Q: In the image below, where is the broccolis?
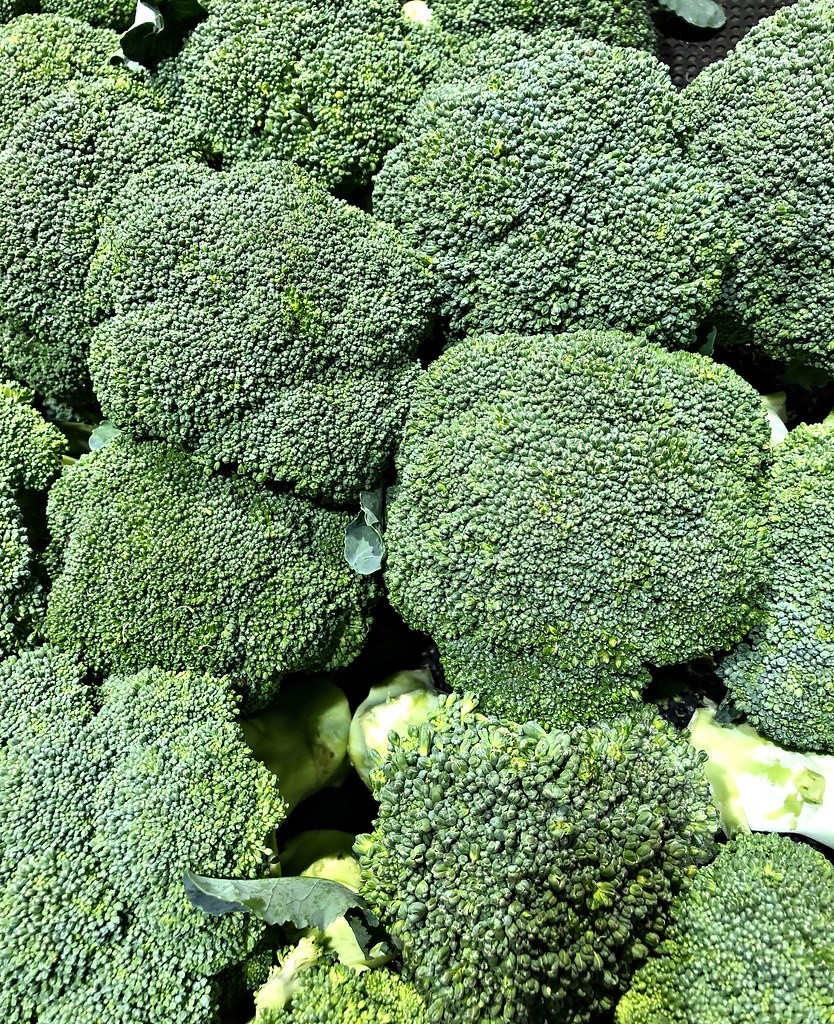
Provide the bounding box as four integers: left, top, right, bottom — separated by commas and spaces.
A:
0, 0, 833, 1024
